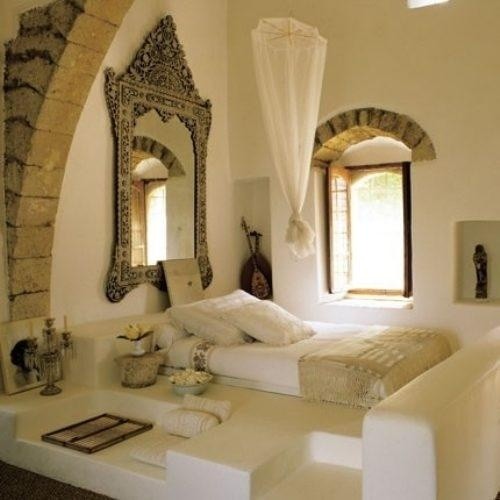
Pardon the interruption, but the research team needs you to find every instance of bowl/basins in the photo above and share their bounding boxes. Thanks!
[169,372,213,396]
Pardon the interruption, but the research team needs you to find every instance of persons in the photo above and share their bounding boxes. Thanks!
[10,339,41,386]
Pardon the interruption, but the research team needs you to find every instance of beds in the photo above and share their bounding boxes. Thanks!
[150,309,458,407]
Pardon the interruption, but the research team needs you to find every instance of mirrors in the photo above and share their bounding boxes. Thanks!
[103,15,212,303]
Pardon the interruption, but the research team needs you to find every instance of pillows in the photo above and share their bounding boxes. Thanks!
[224,299,310,345]
[166,289,264,346]
